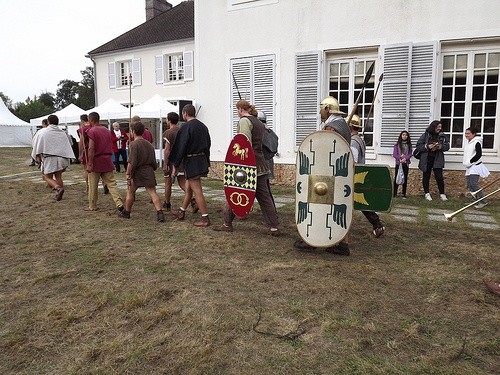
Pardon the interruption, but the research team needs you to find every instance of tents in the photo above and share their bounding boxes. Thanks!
[0,94,181,161]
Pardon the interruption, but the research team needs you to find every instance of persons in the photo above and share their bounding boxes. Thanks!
[416,120,450,201]
[462,127,490,209]
[393,131,412,200]
[29,97,385,256]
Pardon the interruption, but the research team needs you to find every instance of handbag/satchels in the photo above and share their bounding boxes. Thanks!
[396,162,405,185]
[414,133,426,159]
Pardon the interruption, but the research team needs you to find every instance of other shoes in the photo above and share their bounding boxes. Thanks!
[85,207,93,211]
[170,208,185,220]
[475,202,488,208]
[119,209,130,218]
[294,241,316,249]
[191,199,198,214]
[213,224,233,231]
[371,222,384,236]
[193,216,210,226]
[29,164,35,166]
[162,201,171,212]
[157,210,164,222]
[439,194,448,202]
[57,188,64,201]
[117,205,123,214]
[104,184,109,194]
[326,244,350,256]
[267,229,281,236]
[425,193,433,201]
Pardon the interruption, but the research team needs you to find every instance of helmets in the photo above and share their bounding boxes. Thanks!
[320,97,345,115]
[345,114,362,128]
[257,111,266,121]
